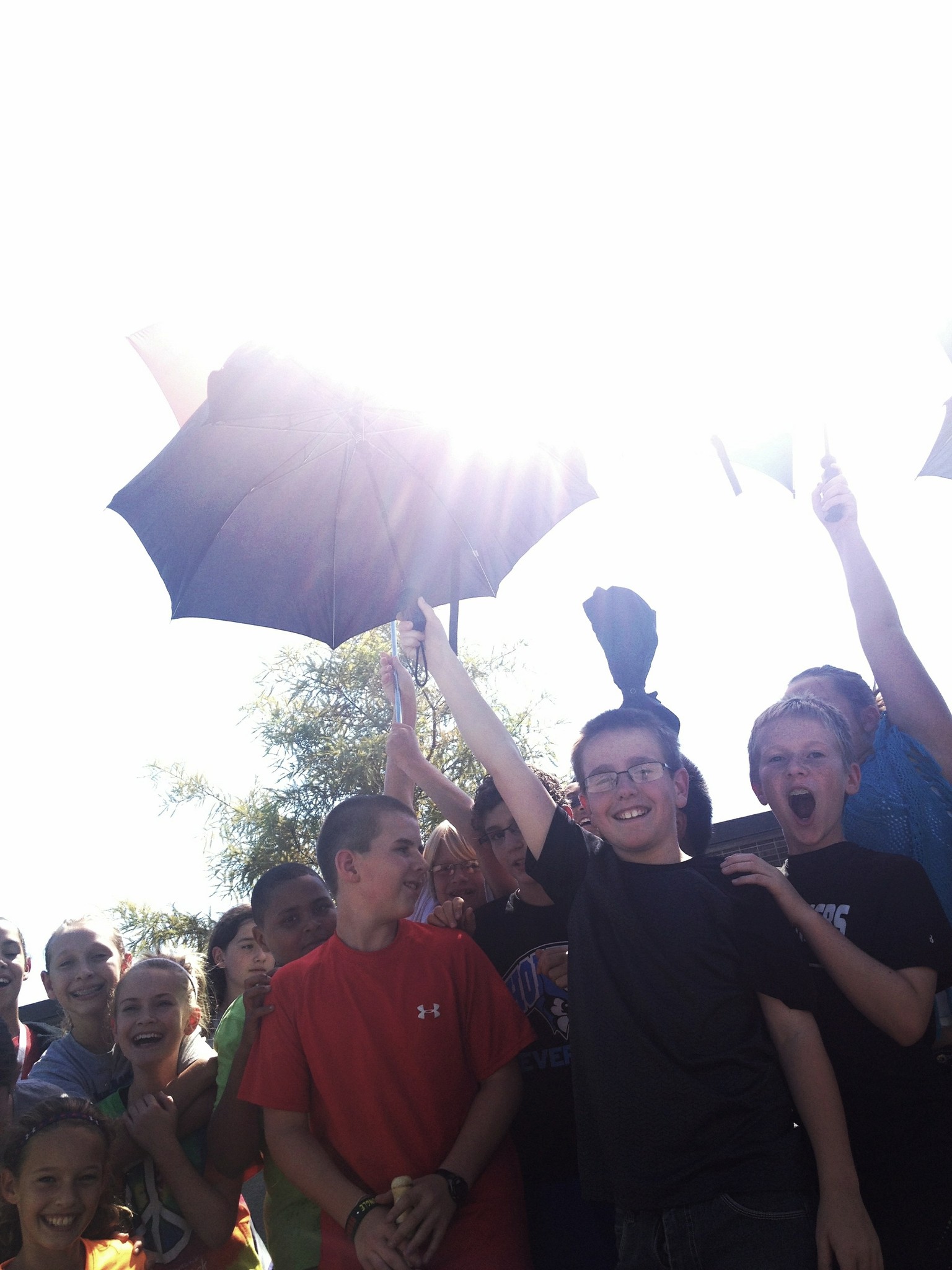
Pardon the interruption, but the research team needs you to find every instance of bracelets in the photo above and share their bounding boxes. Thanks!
[345,1197,378,1242]
[355,1194,368,1205]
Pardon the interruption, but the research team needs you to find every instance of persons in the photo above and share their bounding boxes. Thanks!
[0,457,952,1270]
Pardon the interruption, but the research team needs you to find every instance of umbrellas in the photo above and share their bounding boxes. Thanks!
[115,342,601,688]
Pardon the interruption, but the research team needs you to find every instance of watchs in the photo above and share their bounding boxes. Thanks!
[432,1168,469,1207]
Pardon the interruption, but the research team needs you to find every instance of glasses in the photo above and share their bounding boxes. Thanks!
[567,796,581,808]
[583,760,673,793]
[429,859,481,878]
[478,820,522,850]
